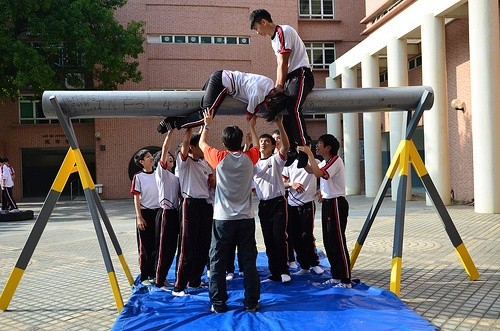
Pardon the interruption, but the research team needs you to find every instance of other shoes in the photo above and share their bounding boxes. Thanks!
[159,116,177,134]
[246,305,259,312]
[211,304,218,313]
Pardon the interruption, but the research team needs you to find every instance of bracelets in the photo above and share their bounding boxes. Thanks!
[204,125,211,130]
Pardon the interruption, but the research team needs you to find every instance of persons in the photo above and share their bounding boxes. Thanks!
[131,130,352,297]
[199,107,260,315]
[249,9,314,168]
[157,70,290,135]
[0,158,19,212]
